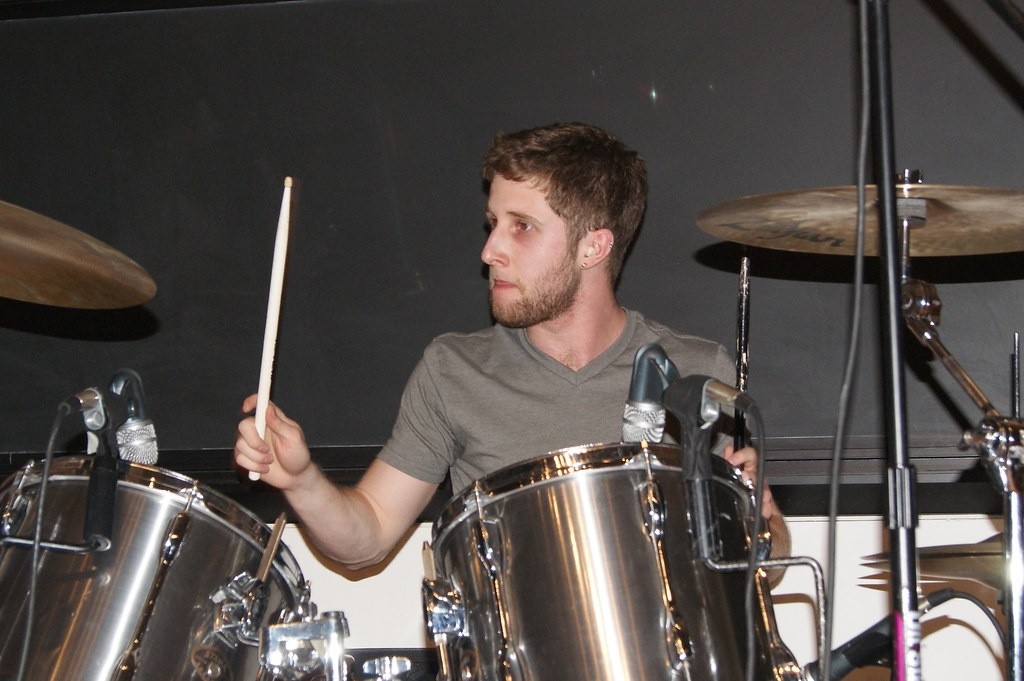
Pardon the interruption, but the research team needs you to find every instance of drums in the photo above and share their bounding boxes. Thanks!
[421,437,804,681]
[0,453,313,681]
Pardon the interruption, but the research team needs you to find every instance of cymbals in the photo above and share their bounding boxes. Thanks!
[859,525,1009,593]
[693,181,1024,259]
[0,195,161,313]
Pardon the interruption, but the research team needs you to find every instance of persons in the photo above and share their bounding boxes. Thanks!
[233,121,791,588]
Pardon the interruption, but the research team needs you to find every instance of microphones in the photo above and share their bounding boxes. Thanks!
[58,367,159,467]
[802,586,955,681]
[622,344,755,444]
